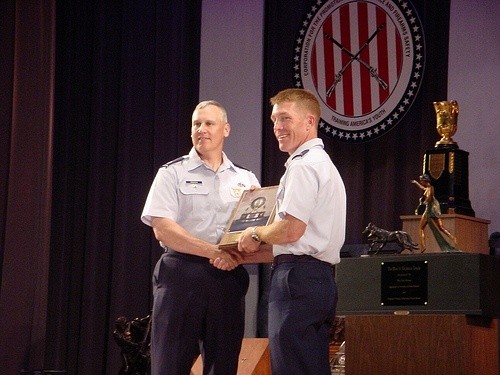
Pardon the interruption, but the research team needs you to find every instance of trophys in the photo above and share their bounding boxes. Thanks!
[413,98,478,216]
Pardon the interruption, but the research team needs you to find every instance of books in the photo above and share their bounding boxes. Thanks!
[218,180,280,251]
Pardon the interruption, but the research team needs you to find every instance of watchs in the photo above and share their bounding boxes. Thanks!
[251,227,260,242]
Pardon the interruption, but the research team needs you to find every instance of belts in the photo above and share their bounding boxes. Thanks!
[163,246,172,253]
[273,254,329,267]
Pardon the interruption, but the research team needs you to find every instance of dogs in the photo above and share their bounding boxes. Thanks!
[361,222,421,255]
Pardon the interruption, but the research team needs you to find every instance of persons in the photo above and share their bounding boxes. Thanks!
[217,89,345,375]
[409,173,460,255]
[139,102,251,375]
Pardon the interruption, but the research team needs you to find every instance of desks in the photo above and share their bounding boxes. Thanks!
[344,314,500,375]
